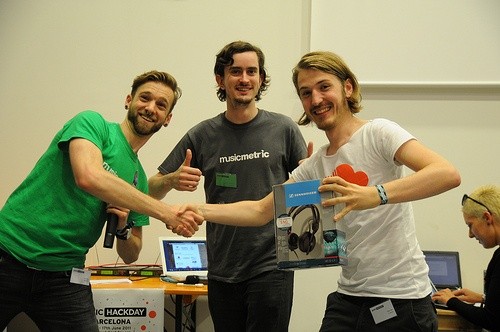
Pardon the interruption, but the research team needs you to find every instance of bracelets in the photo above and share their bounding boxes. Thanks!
[374,184,388,206]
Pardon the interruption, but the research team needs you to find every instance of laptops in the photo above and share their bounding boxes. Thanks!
[158,236,209,282]
[422,251,475,310]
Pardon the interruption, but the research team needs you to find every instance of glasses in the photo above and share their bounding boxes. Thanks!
[462,194,492,215]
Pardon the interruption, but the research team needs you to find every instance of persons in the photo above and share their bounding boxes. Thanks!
[166,51,463,332]
[0,69,205,332]
[146,40,315,332]
[431,185,500,332]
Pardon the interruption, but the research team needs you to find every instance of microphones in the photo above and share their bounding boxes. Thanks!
[103,206,120,248]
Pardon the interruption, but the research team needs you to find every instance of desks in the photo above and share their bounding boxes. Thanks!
[90,276,208,332]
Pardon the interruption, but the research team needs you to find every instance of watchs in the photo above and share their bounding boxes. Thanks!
[115,223,133,241]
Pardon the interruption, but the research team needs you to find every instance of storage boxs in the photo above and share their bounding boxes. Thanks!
[272,179,347,271]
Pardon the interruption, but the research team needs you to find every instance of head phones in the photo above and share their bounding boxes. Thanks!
[287,203,319,256]
